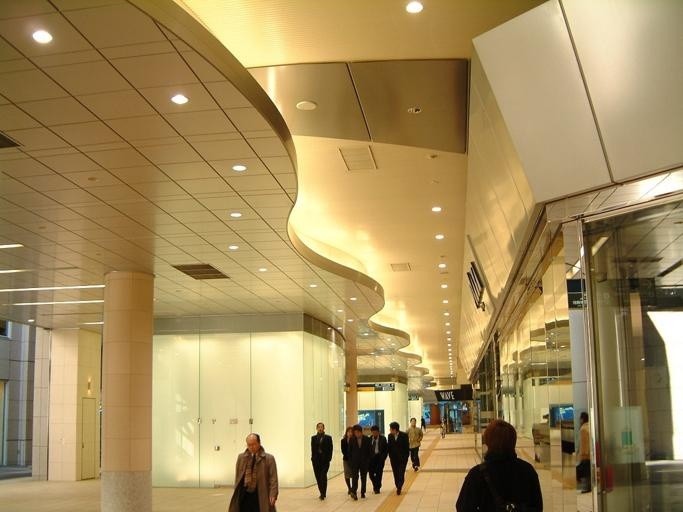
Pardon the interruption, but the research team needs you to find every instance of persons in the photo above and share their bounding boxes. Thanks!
[421,416,426,430]
[341,427,354,494]
[311,423,333,500]
[228,433,278,512]
[577,412,591,493]
[369,425,388,494]
[348,425,371,500]
[388,422,409,495]
[406,418,423,471]
[456,419,543,512]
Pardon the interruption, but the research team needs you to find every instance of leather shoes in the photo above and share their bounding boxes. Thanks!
[348,488,380,501]
[396,488,400,495]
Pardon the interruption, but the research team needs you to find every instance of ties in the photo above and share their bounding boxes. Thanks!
[251,454,256,474]
[372,439,375,453]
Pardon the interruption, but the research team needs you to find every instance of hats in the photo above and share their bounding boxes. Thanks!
[484,419,516,449]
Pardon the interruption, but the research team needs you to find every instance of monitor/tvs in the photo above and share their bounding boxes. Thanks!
[550,405,574,426]
[358,410,384,435]
